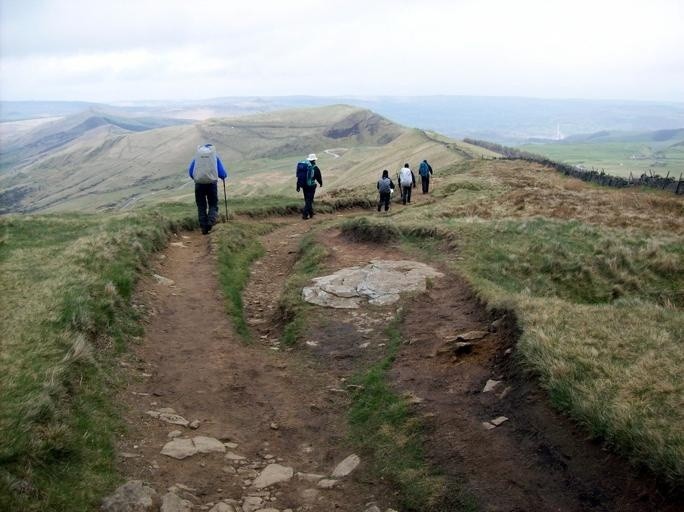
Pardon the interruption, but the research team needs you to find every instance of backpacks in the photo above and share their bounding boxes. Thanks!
[378,177,390,195]
[192,144,218,186]
[294,160,318,188]
[398,168,412,188]
[418,161,430,177]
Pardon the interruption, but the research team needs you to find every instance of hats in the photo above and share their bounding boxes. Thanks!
[305,153,319,161]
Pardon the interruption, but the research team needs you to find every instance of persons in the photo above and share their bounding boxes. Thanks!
[418,159,433,195]
[376,170,395,212]
[187,143,227,235]
[397,163,416,205]
[295,153,323,220]
